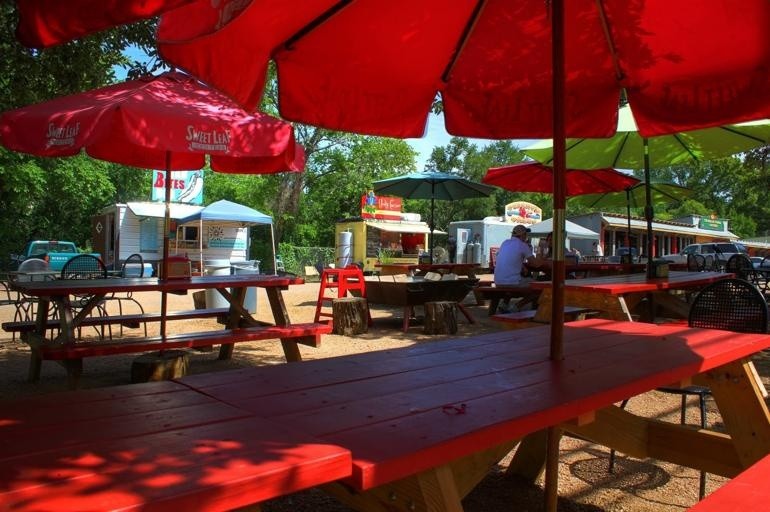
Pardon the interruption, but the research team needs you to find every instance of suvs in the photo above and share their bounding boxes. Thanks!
[662,243,747,271]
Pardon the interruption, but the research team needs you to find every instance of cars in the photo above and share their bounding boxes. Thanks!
[748,257,770,271]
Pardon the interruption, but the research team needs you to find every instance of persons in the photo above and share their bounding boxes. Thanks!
[530,230,578,311]
[494,224,546,313]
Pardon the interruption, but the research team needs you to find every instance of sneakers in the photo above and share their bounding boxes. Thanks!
[499,302,519,313]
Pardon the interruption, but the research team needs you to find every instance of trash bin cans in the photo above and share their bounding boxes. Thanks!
[231,260,261,314]
[205,259,231,309]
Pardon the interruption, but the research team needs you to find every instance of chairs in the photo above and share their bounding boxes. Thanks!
[0,252,147,341]
[686,251,770,303]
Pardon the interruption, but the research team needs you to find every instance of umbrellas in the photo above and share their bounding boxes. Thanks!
[480,159,641,196]
[19,0,770,512]
[567,180,697,262]
[371,169,497,264]
[1,64,308,353]
[517,101,770,325]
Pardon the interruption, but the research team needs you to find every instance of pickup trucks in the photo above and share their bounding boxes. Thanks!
[8,239,102,276]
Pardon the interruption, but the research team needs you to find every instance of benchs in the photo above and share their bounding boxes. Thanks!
[0,263,769,512]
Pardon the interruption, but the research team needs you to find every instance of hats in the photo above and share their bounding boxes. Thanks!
[512,225,531,236]
